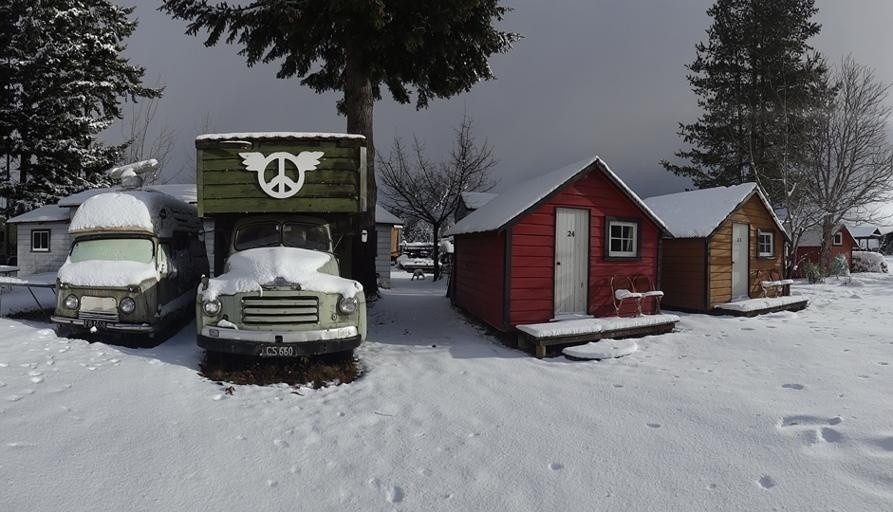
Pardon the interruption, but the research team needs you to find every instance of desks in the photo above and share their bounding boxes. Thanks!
[0,278,56,323]
[0,265,20,290]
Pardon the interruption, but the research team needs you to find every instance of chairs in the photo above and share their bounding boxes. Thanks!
[757,270,782,299]
[771,270,794,298]
[611,273,646,319]
[634,272,664,317]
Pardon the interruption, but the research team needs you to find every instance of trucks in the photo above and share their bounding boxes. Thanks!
[194,131,368,380]
[49,188,209,345]
